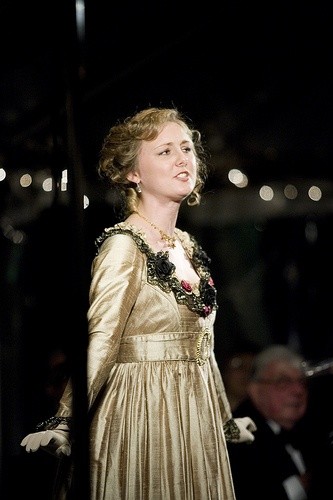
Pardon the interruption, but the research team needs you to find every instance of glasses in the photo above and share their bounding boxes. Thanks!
[250,373,308,387]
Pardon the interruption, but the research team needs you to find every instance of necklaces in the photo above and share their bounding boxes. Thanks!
[134,210,176,248]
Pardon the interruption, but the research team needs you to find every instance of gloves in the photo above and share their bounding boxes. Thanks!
[20,430,71,457]
[222,417,257,443]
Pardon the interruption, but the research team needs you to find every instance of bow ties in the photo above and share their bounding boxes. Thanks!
[280,427,304,441]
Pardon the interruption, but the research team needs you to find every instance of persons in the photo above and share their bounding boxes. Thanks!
[20,109,258,500]
[223,345,326,500]
[218,342,260,412]
